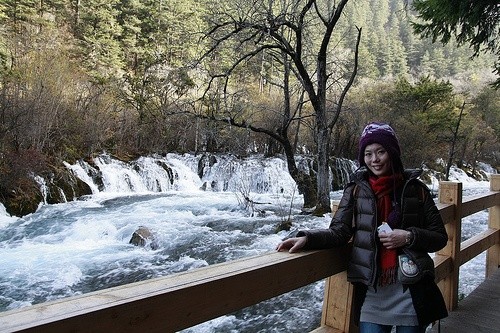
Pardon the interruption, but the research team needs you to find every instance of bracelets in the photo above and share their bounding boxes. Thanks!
[405,232,412,245]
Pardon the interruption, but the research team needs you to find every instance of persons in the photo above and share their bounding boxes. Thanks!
[276,123,448,333]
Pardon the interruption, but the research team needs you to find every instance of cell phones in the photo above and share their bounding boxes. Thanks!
[377,223,392,235]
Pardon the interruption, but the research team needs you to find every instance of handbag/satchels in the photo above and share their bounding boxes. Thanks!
[397,247,436,285]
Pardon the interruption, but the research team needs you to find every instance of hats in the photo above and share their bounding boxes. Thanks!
[355,120,405,222]
